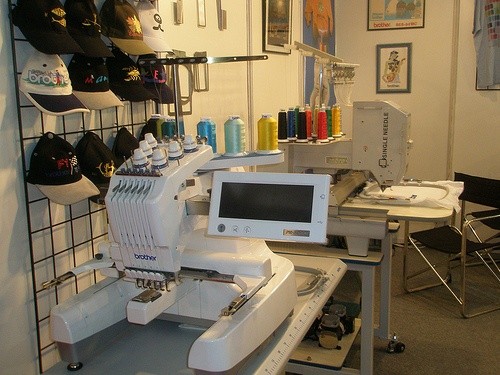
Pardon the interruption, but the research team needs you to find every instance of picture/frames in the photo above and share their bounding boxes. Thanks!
[367,0,425,31]
[262,0,292,54]
[376,42,412,93]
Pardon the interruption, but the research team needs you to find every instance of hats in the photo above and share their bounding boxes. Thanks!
[7,0,175,205]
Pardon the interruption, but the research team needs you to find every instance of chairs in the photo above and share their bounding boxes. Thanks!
[398,170,500,318]
